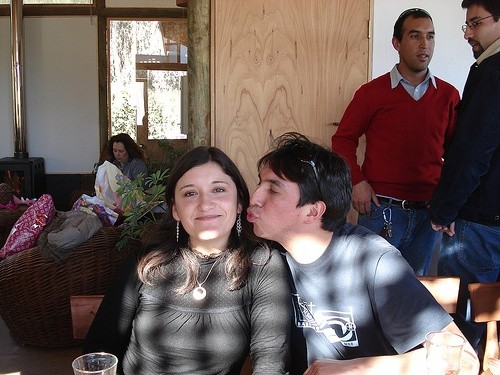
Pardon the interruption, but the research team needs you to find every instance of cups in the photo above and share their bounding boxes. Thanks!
[72,352,118,375]
[426,329,465,374]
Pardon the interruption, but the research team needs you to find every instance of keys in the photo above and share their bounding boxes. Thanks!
[382,222,393,239]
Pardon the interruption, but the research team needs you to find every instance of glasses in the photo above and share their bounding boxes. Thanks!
[280,145,324,203]
[461,13,500,33]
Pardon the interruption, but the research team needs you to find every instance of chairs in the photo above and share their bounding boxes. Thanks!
[0,225,142,348]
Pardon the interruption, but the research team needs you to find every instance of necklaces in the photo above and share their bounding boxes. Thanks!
[184,247,225,303]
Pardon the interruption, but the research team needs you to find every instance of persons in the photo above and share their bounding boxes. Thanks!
[78,144,297,374]
[99,133,148,186]
[331,8,460,277]
[244,130,481,375]
[430,0,500,351]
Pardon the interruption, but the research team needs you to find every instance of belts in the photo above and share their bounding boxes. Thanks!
[376,197,430,210]
